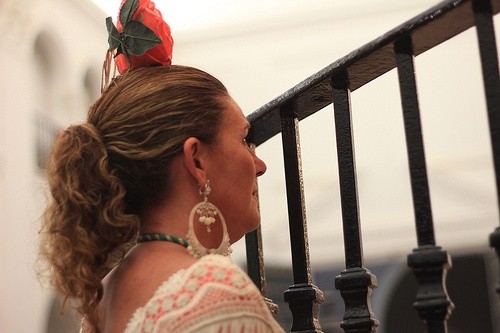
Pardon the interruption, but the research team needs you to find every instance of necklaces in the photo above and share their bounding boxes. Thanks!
[135,232,190,249]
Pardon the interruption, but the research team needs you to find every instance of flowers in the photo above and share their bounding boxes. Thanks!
[105,0,174,74]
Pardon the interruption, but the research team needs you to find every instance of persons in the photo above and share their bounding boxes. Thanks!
[38,62,284,332]
